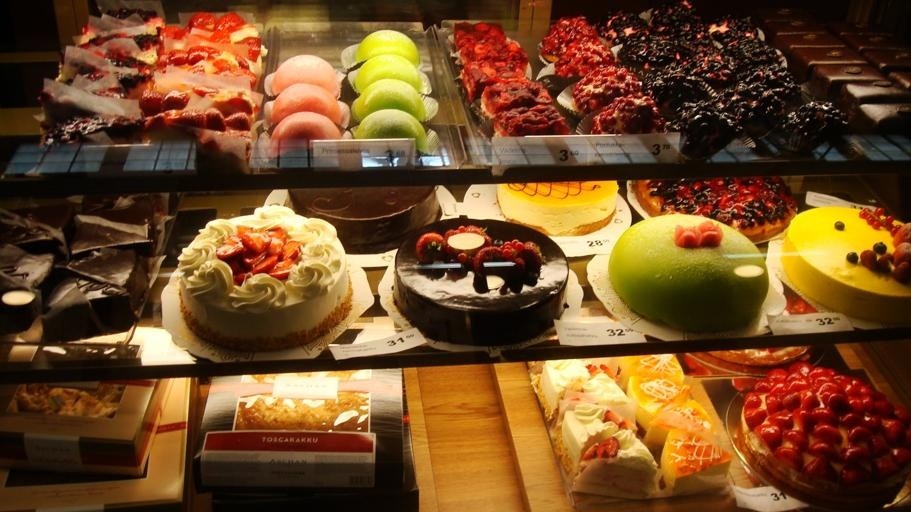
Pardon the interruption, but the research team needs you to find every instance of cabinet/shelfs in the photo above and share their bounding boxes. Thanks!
[0,0,911,512]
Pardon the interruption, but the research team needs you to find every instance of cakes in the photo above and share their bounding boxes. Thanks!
[780,206,910,324]
[750,0,911,134]
[735,362,910,509]
[354,30,420,67]
[527,353,733,499]
[288,185,441,254]
[270,83,343,126]
[355,109,427,151]
[271,55,337,96]
[177,204,353,353]
[608,213,768,333]
[355,79,427,122]
[635,175,796,243]
[354,55,423,92]
[32,7,268,169]
[688,343,812,377]
[496,180,619,236]
[454,15,666,136]
[0,192,163,344]
[268,111,341,159]
[393,219,570,346]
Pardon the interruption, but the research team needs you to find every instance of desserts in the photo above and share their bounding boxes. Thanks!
[587,0,849,162]
